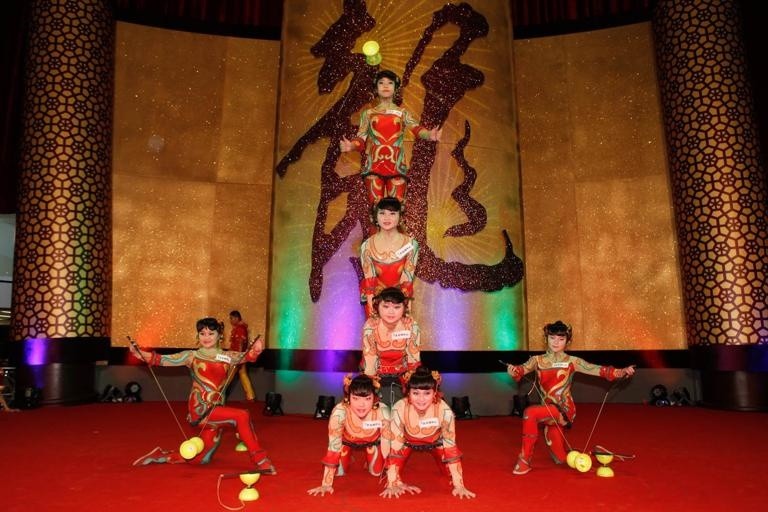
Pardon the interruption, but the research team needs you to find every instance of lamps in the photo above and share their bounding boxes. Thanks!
[313,394,336,418]
[261,392,285,417]
[97,382,142,403]
[451,394,528,420]
[648,385,689,407]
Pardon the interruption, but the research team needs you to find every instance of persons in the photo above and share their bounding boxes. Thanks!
[357,195,422,321]
[355,285,422,408]
[226,308,255,403]
[504,318,638,474]
[336,67,445,238]
[304,373,423,500]
[379,364,478,500]
[126,315,280,477]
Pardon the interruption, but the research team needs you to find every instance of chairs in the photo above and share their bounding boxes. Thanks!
[0,368,11,412]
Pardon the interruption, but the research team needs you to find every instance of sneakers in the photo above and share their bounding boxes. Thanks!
[255,457,278,476]
[595,445,625,463]
[132,446,168,467]
[512,459,533,475]
